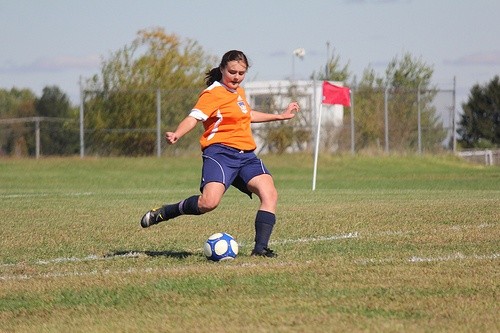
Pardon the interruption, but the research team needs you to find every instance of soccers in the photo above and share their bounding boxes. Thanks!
[205,233,239,262]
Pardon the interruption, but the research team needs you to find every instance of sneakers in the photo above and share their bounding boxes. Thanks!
[251,243,278,259]
[141,204,169,228]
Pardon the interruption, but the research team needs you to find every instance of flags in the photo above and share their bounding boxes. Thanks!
[321,80,351,107]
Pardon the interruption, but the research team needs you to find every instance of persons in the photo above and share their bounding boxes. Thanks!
[141,50,300,258]
